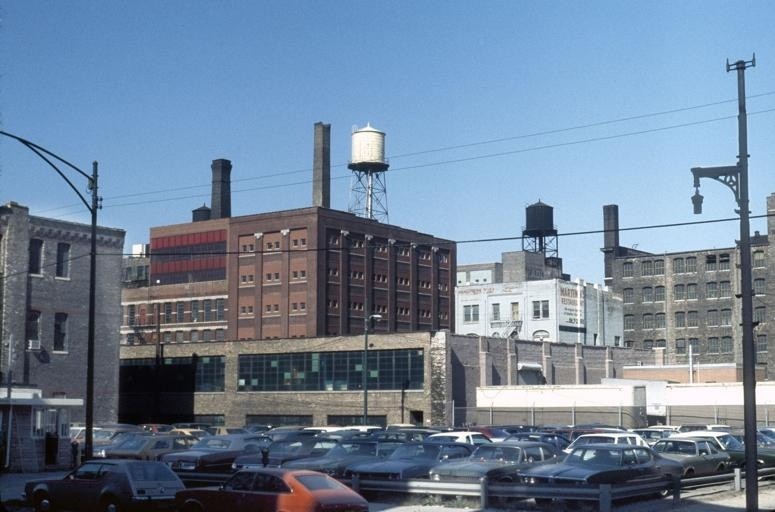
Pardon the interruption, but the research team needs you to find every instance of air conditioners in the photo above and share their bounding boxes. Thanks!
[27,340,41,350]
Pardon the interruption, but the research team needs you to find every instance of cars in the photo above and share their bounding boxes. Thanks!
[173,467,370,512]
[515,443,686,509]
[69,422,775,502]
[23,459,188,512]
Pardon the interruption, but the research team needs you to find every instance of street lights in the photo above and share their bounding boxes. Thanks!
[1,132,104,459]
[689,163,759,511]
[364,313,384,424]
